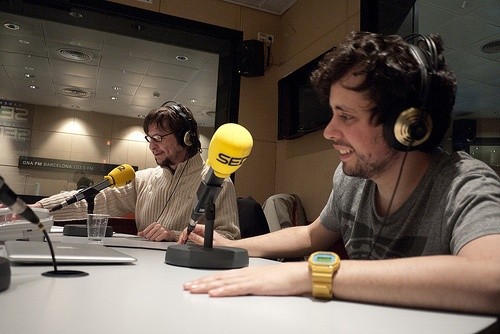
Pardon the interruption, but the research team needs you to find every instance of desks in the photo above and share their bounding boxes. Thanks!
[0,233,497,334]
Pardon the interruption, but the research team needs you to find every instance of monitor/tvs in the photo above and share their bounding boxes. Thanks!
[277,47,337,141]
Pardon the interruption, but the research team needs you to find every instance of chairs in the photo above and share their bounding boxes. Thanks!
[238,196,271,238]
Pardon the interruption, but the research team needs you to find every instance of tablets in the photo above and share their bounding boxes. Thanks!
[4,241,137,263]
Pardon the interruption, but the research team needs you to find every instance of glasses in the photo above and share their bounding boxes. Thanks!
[144,131,177,142]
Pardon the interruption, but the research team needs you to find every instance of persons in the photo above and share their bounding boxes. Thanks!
[0,101,240,242]
[75,177,94,190]
[176,31,500,317]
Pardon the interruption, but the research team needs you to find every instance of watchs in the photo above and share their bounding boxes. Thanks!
[308,252,340,300]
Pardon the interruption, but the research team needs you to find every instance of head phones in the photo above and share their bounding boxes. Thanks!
[163,101,199,149]
[381,32,438,152]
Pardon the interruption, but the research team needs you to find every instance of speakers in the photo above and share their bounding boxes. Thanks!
[231,40,265,78]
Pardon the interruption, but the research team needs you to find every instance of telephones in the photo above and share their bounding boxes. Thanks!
[0,207,54,242]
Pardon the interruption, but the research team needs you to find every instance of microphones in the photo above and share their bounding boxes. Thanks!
[0,176,45,230]
[187,123,254,233]
[51,164,135,212]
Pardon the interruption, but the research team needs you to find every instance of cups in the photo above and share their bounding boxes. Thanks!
[85,213,111,242]
